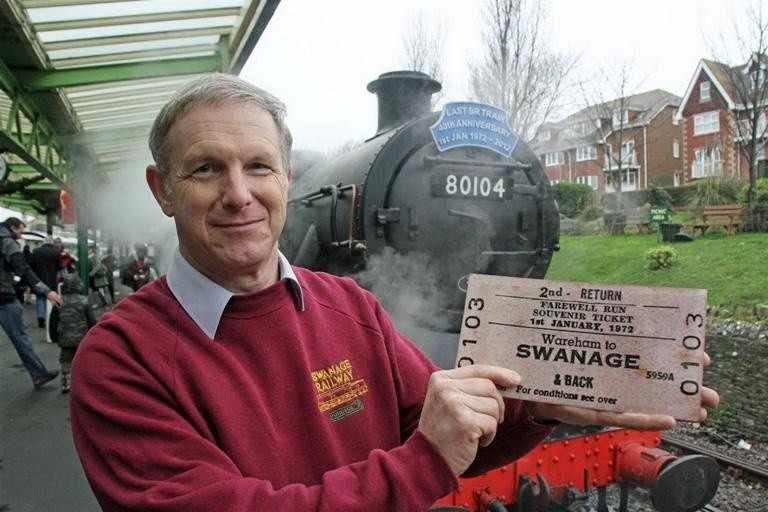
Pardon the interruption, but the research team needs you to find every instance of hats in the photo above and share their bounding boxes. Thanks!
[44,235,54,243]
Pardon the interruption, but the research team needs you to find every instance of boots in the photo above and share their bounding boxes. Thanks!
[61,371,71,393]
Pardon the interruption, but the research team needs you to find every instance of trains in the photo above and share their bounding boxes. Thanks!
[278,70,721,512]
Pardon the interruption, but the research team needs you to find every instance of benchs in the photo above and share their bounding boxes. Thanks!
[604,202,745,237]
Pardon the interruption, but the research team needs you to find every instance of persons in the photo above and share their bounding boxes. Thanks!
[48,274,97,393]
[69,71,722,510]
[1,217,65,386]
[14,238,159,343]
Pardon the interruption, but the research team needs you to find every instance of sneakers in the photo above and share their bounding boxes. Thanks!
[33,369,60,387]
[91,301,118,310]
[37,318,45,328]
